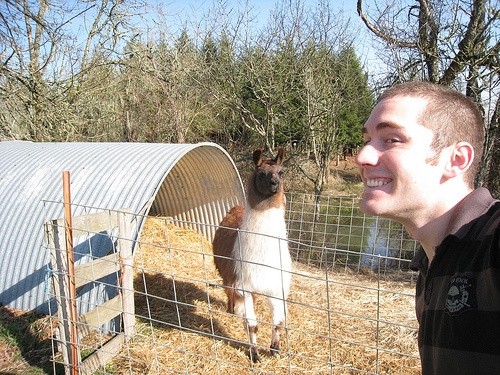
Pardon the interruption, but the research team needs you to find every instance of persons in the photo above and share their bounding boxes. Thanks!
[354,81,500,375]
